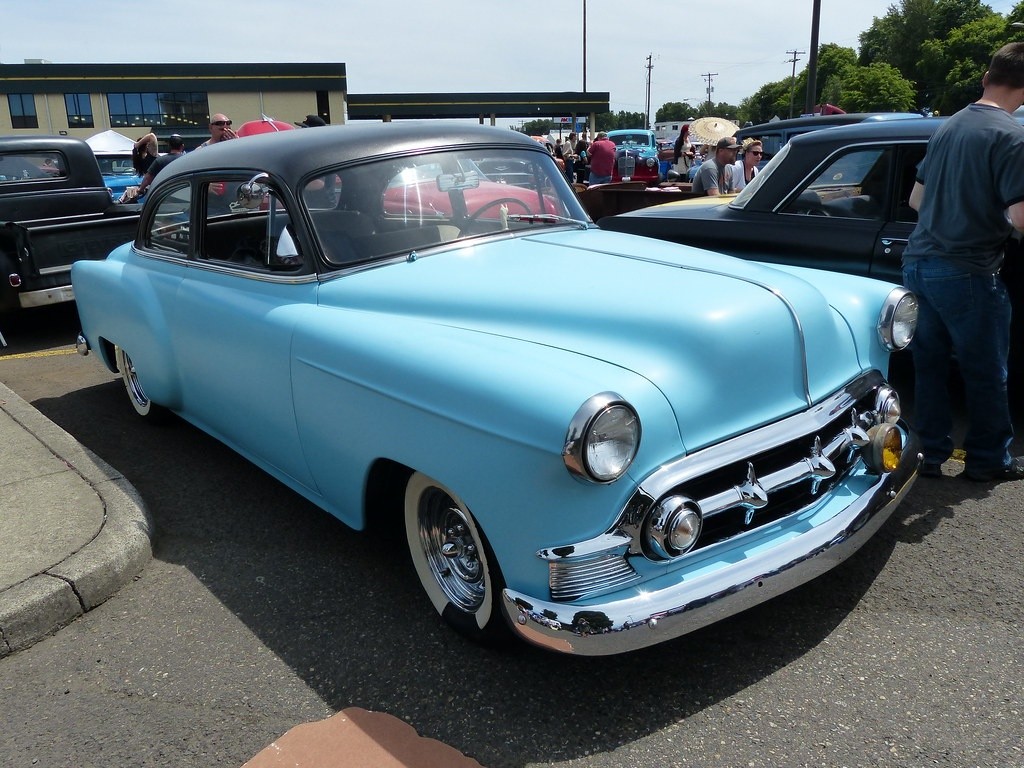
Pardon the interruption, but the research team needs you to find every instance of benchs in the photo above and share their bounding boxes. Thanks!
[178,209,375,264]
[796,189,921,222]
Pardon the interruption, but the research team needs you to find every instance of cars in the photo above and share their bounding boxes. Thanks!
[575,113,1024,287]
[0,120,923,655]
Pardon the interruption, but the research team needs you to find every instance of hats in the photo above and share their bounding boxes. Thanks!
[717,138,741,149]
[295,114,326,128]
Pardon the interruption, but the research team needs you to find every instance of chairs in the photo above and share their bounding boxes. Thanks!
[355,225,466,259]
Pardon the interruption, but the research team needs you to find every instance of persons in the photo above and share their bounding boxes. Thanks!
[293,114,335,209]
[692,136,741,195]
[724,138,763,193]
[546,132,615,186]
[45,158,52,165]
[700,144,715,160]
[195,113,239,216]
[107,132,184,203]
[901,43,1024,482]
[674,124,694,182]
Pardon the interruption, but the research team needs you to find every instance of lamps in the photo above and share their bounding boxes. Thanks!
[59,131,67,135]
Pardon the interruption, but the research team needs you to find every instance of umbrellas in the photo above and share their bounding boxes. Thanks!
[689,117,741,146]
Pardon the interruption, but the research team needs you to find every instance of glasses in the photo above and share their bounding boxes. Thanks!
[748,151,764,156]
[211,120,233,126]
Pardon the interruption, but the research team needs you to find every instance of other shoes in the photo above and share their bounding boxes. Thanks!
[966,455,1023,480]
[917,461,942,475]
